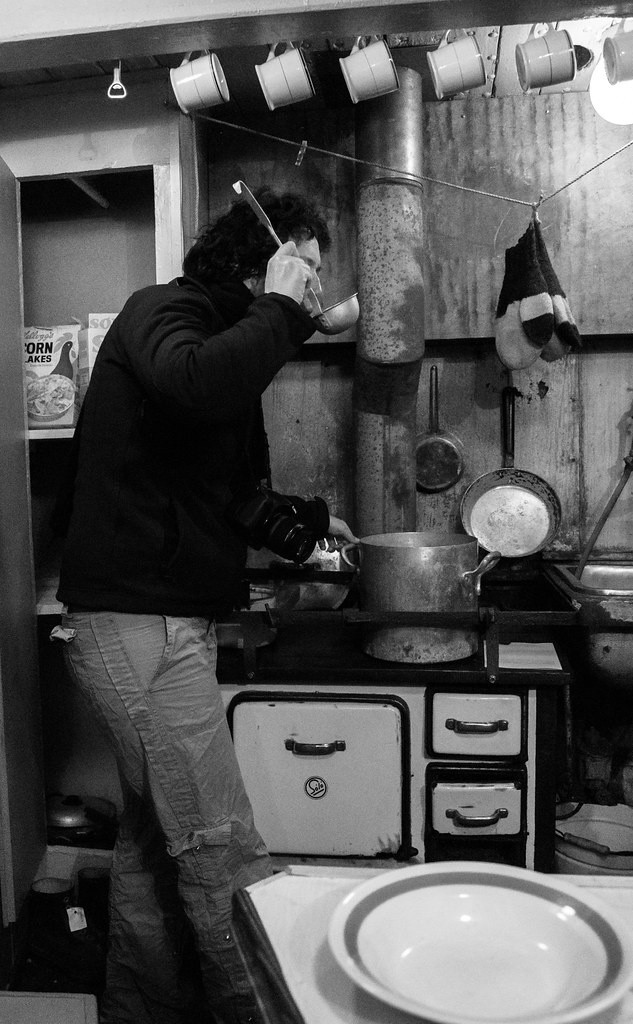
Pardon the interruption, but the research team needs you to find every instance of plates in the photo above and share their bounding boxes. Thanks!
[328,857,633,1024]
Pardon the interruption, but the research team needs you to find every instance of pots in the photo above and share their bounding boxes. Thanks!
[459,386,563,559]
[231,585,280,650]
[338,529,502,665]
[414,365,466,492]
[252,536,351,608]
[45,791,126,844]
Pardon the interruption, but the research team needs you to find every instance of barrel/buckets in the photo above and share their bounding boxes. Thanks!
[553,799,633,877]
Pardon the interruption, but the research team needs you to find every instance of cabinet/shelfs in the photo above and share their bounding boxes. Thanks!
[29,427,79,614]
[217,683,537,865]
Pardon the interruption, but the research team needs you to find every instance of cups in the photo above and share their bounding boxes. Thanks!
[337,30,401,104]
[426,27,487,100]
[169,44,231,114]
[602,17,633,86]
[253,38,317,112]
[514,22,578,92]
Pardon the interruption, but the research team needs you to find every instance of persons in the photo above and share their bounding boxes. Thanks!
[56,190,361,1024]
